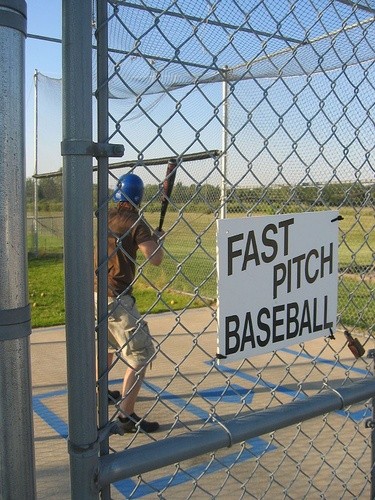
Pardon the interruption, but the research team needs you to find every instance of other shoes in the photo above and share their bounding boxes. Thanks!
[108,390,120,404]
[116,414,159,433]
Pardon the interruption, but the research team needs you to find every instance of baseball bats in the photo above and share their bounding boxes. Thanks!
[158,159,177,232]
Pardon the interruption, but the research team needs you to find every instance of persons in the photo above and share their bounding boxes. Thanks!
[91,174,166,433]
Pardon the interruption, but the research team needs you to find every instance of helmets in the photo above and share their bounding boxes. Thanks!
[112,174,144,203]
